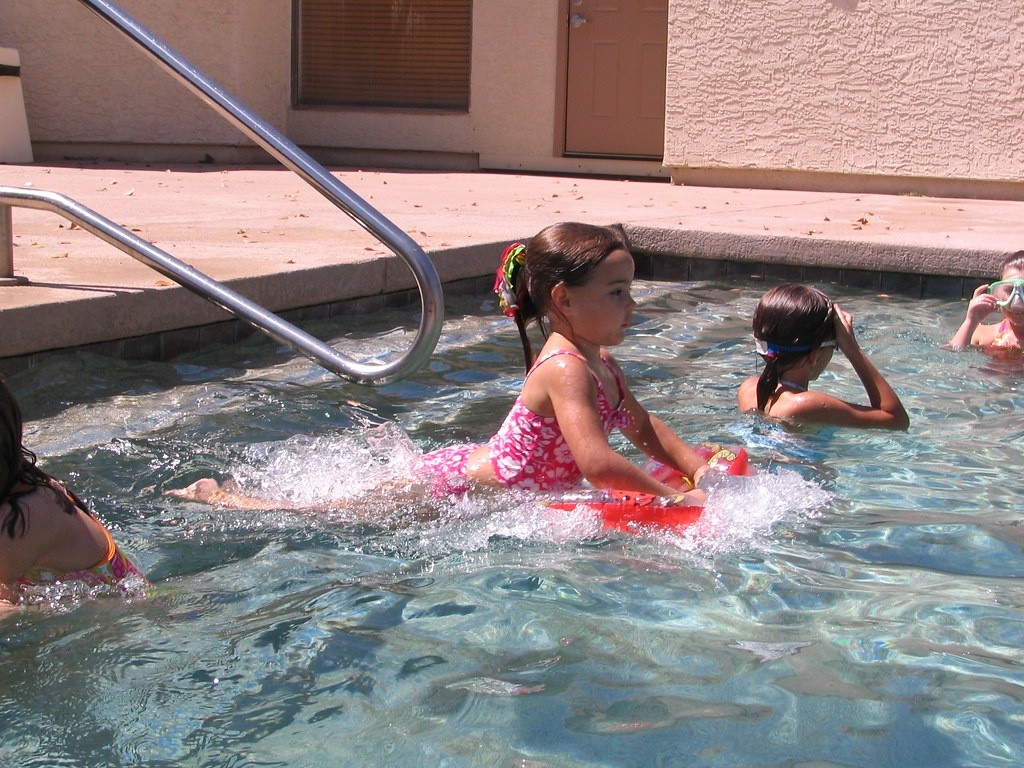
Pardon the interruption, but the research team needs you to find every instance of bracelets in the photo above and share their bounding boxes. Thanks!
[692,466,711,487]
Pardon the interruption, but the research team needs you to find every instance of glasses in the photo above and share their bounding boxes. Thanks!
[986,280,1024,309]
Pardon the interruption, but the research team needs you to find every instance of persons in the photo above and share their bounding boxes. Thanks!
[0,371,165,602]
[942,249,1024,381]
[164,222,721,530]
[708,282,910,540]
